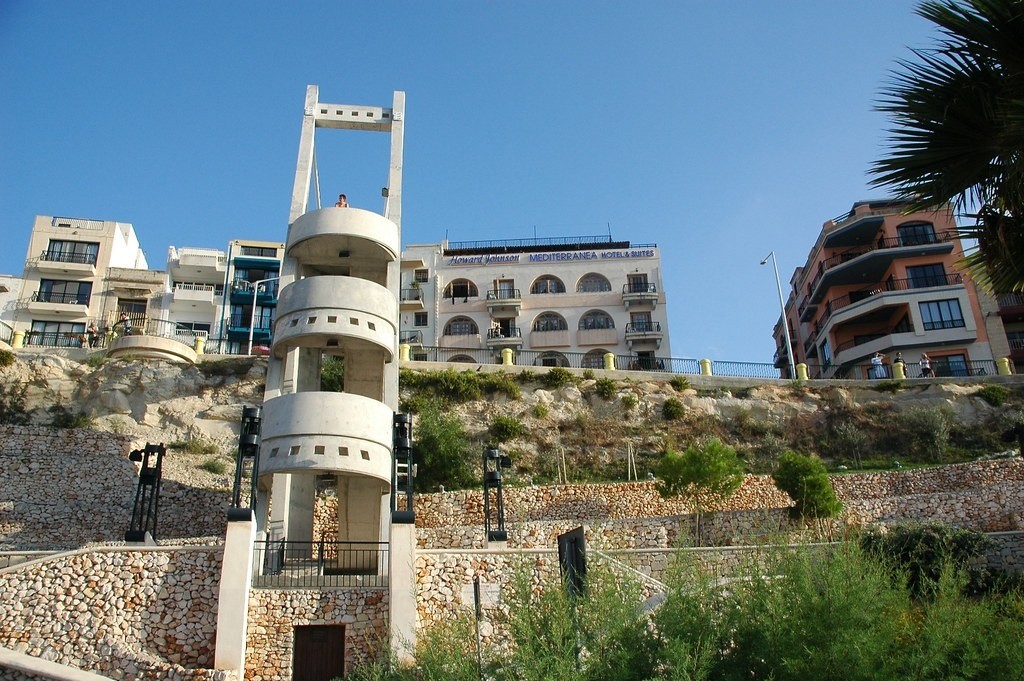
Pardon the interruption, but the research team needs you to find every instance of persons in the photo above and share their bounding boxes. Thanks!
[102,326,117,348]
[871,352,886,379]
[628,360,637,370]
[258,282,266,293]
[87,322,98,348]
[335,194,349,208]
[919,353,938,378]
[121,313,132,336]
[894,352,907,378]
[653,360,665,372]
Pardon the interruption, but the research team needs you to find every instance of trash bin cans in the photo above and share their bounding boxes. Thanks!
[195,338,206,355]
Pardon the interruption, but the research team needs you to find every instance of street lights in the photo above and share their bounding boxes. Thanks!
[759,250,797,379]
